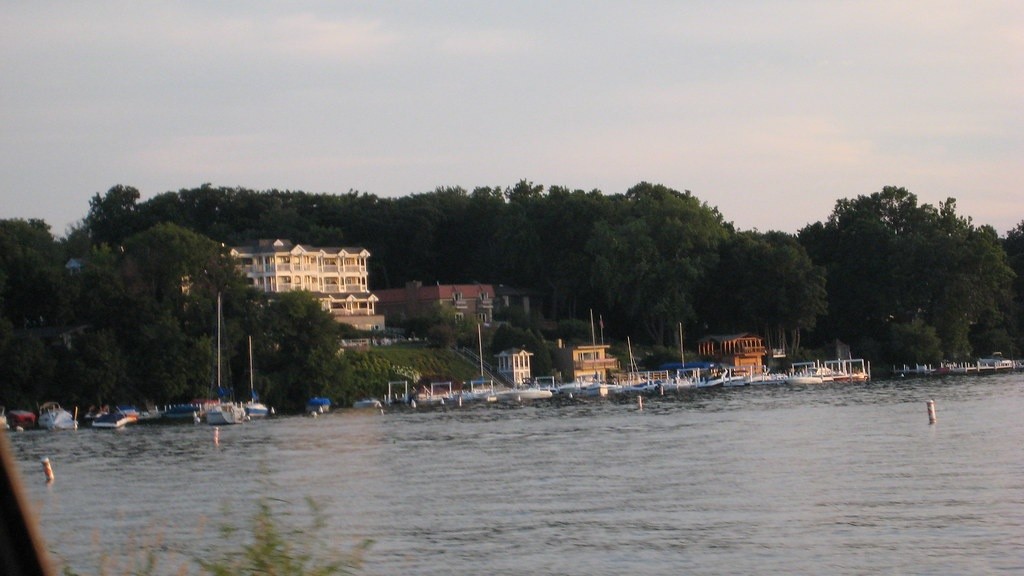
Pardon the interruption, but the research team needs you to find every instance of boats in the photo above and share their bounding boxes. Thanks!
[39,402,77,430]
[93,416,138,429]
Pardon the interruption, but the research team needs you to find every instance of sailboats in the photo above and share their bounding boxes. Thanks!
[354,309,873,410]
[206,292,267,424]
